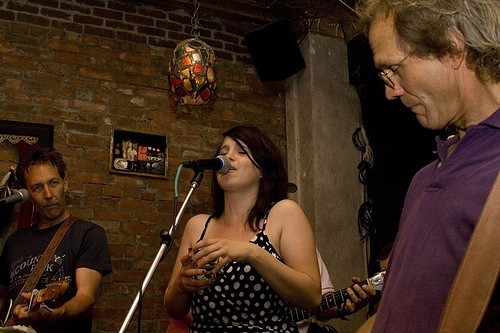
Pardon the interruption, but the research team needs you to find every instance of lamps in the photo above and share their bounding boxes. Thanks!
[168,0,217,113]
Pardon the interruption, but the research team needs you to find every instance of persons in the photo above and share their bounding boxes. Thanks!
[356,0,500,333]
[296,247,376,333]
[165,125,322,333]
[0,148,113,333]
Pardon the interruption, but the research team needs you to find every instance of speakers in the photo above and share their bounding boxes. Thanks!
[243,20,306,83]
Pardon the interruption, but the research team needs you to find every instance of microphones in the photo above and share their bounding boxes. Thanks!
[0,189,30,203]
[180,155,230,175]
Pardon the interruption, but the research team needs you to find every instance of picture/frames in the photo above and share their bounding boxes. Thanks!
[107,128,171,178]
[0,120,55,251]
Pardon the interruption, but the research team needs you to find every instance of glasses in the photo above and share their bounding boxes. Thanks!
[378,49,418,88]
